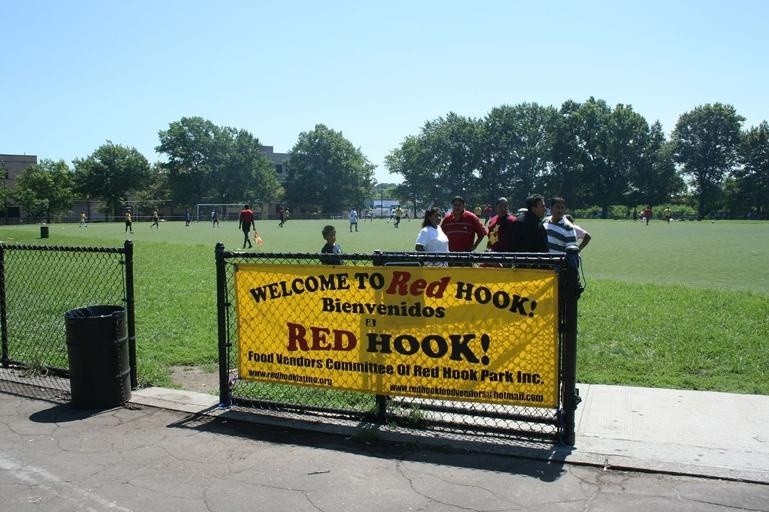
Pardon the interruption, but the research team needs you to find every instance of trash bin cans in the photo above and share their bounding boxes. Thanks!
[41,227,49,238]
[63,305,132,409]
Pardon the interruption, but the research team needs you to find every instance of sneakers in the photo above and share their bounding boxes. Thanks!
[248,245,253,249]
[243,247,246,249]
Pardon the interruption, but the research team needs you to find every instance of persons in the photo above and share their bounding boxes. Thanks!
[321,225,343,265]
[415,194,591,299]
[79,211,87,228]
[349,205,411,232]
[212,208,219,227]
[150,209,159,230]
[632,207,653,226]
[125,209,133,233]
[186,207,192,226]
[285,208,290,221]
[279,207,287,227]
[239,205,256,248]
[664,207,673,223]
